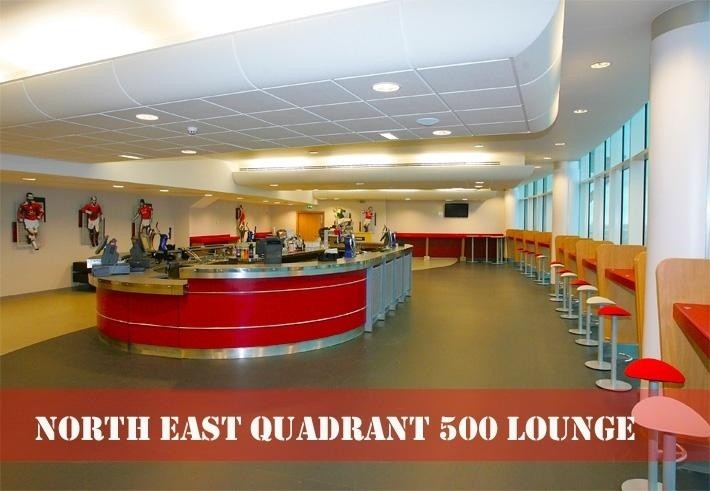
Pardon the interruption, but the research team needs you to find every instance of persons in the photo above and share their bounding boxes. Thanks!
[80,196,102,247]
[132,199,153,235]
[239,204,246,225]
[362,207,375,232]
[17,192,44,250]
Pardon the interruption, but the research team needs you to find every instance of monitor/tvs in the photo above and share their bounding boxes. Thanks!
[445,203,468,217]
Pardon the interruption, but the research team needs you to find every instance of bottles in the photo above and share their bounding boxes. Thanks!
[236,245,254,262]
[301,240,305,252]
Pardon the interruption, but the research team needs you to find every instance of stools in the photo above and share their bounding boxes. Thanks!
[469,234,708,491]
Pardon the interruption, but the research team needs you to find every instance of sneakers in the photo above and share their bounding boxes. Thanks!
[27,236,31,244]
[33,247,39,251]
[91,240,99,247]
